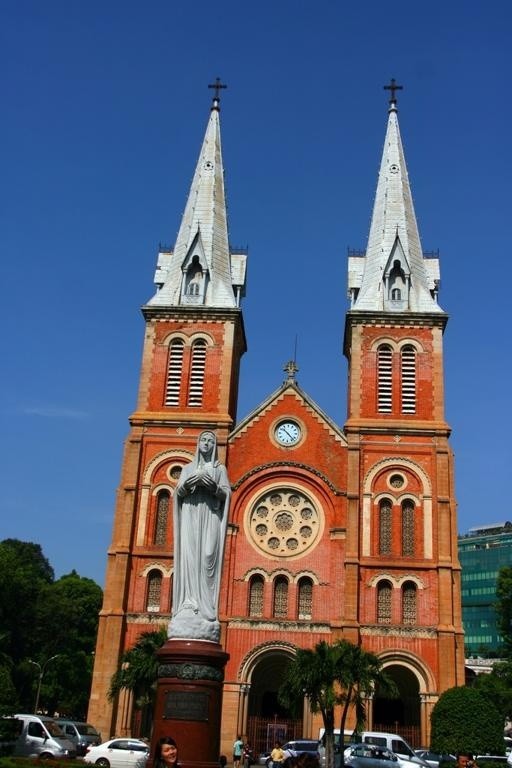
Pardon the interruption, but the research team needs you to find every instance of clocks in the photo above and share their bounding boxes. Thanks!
[272,417,303,447]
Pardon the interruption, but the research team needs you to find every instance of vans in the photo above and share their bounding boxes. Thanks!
[259,740,319,768]
[2,712,102,761]
[317,728,431,768]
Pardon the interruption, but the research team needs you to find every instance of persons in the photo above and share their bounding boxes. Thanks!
[454,750,479,768]
[151,736,181,768]
[271,742,284,768]
[283,751,321,768]
[37,707,60,717]
[233,736,257,768]
[167,430,230,642]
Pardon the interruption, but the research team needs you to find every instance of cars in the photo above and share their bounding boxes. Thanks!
[343,744,426,768]
[83,737,151,768]
[414,749,458,768]
